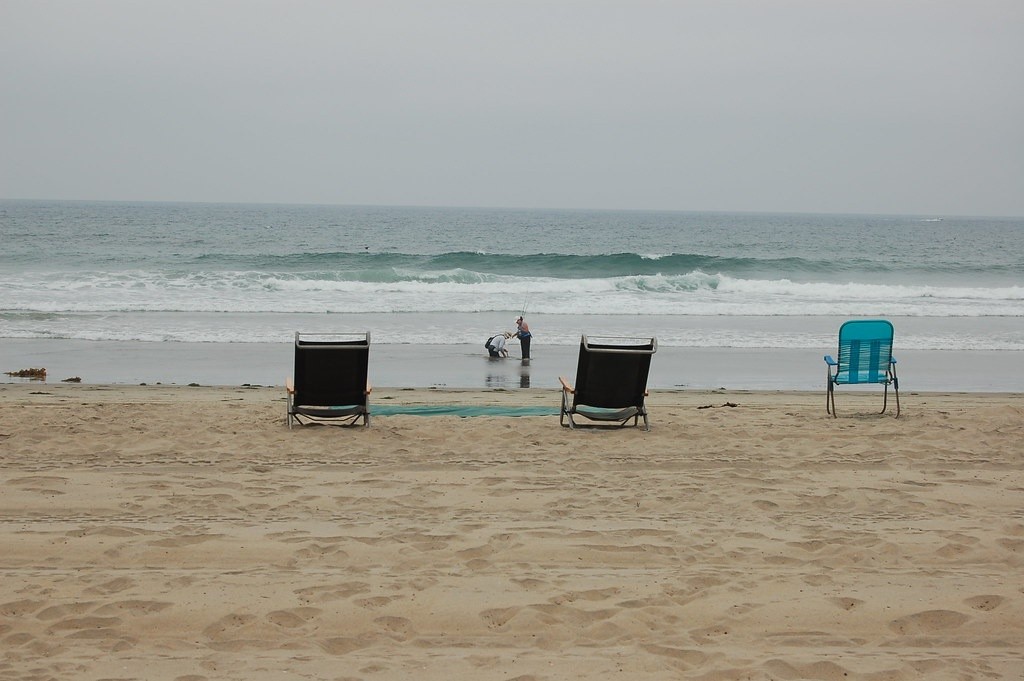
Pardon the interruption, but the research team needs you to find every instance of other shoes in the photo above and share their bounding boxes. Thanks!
[524,358,529,360]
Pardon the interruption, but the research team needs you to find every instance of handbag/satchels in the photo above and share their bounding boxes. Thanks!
[484,336,493,348]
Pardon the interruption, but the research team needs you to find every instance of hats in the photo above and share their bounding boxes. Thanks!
[505,330,513,336]
[513,315,520,323]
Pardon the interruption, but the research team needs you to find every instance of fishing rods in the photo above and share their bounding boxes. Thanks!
[518,274,532,337]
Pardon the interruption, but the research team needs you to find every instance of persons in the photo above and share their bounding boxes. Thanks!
[488,331,511,358]
[512,316,531,359]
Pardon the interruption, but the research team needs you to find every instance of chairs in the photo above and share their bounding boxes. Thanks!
[558,334,658,432]
[824,318,900,419]
[287,331,372,430]
